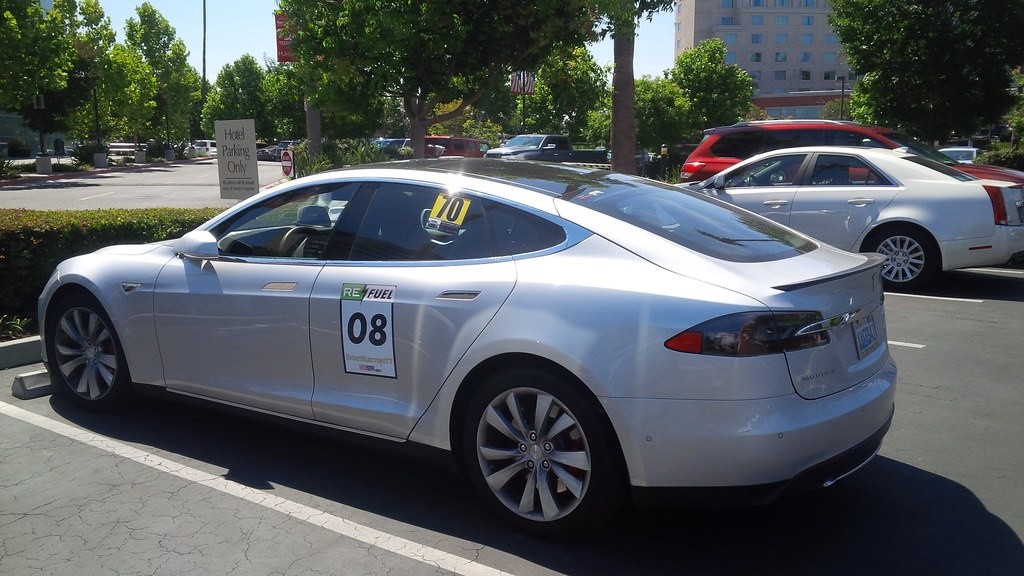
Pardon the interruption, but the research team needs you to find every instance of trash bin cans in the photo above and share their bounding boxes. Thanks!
[52,138,65,155]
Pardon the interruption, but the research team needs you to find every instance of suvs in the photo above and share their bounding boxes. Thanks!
[680,119,1024,187]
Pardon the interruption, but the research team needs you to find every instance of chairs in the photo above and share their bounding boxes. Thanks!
[371,191,429,260]
[827,158,852,184]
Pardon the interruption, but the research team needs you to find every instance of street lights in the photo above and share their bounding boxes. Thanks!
[835,74,846,120]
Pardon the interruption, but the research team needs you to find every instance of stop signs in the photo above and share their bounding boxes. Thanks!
[281,152,293,175]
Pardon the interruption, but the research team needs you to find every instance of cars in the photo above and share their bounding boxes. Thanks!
[37,159,900,547]
[256,141,302,162]
[666,145,1024,292]
[936,136,991,164]
[192,138,217,156]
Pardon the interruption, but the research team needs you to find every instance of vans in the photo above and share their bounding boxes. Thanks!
[372,135,491,161]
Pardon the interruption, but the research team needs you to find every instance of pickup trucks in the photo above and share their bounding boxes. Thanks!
[483,134,608,165]
